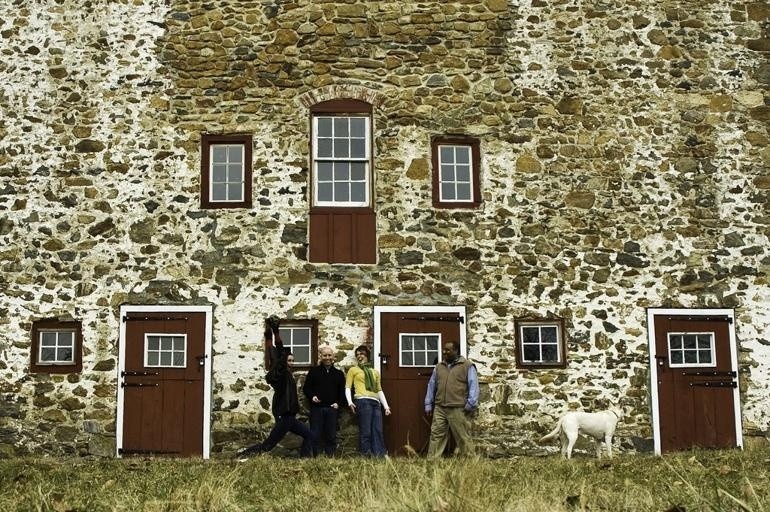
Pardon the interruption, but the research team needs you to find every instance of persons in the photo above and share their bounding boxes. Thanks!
[423,339,480,460]
[236,314,317,459]
[345,345,393,457]
[302,346,345,456]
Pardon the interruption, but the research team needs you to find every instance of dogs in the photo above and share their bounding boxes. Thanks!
[538,401,631,461]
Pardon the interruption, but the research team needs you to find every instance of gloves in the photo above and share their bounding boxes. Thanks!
[265,317,281,333]
